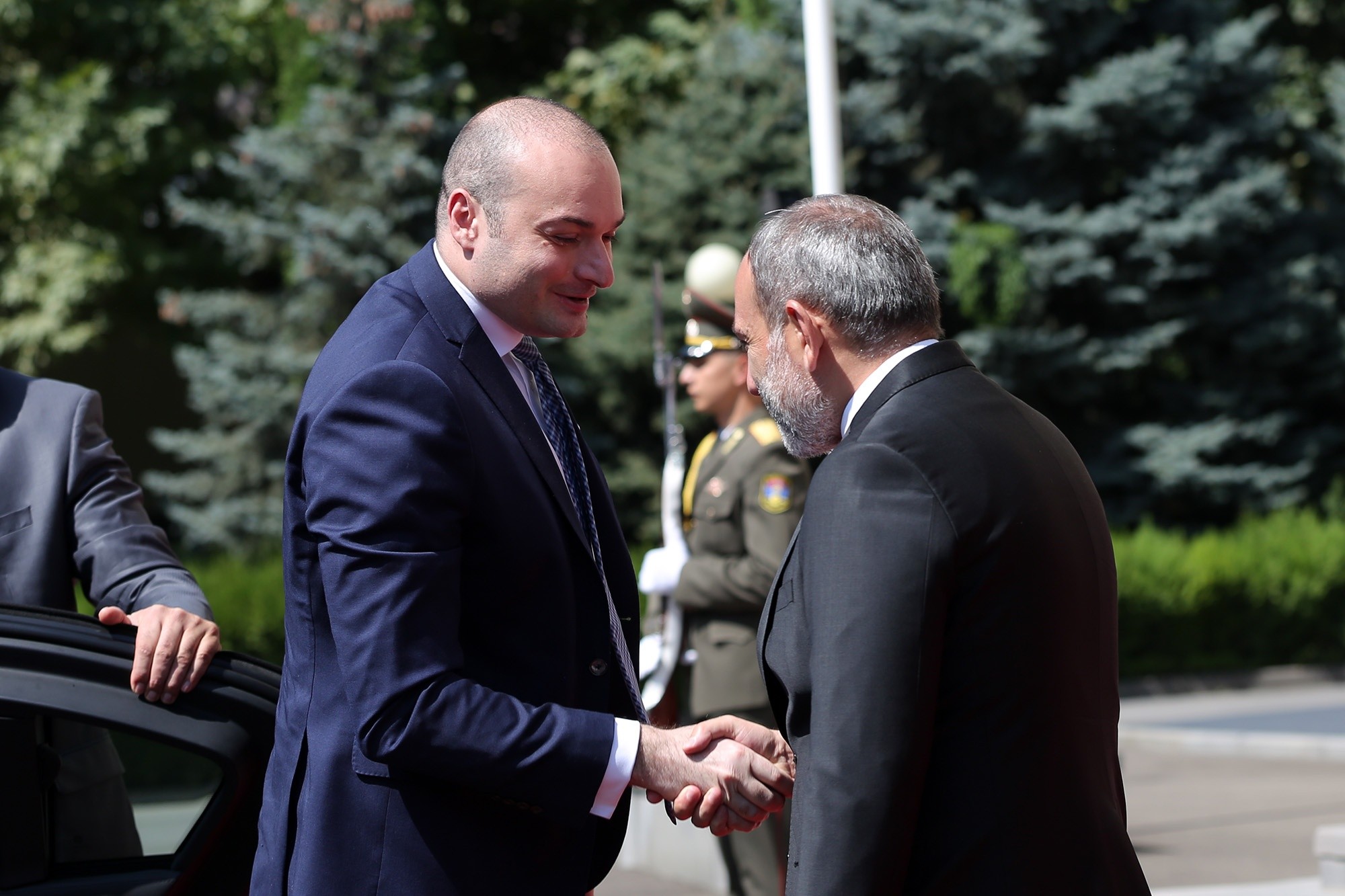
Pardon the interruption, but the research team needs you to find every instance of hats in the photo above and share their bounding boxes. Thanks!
[679,290,749,359]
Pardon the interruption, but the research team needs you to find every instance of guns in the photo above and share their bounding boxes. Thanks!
[637,259,689,710]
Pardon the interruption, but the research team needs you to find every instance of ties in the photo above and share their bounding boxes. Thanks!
[511,335,676,825]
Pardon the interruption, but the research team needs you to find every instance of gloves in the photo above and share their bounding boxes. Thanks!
[639,634,697,679]
[638,546,689,596]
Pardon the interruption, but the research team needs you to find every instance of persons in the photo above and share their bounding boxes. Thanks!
[644,192,1155,896]
[0,367,221,704]
[630,243,817,896]
[246,94,793,896]
